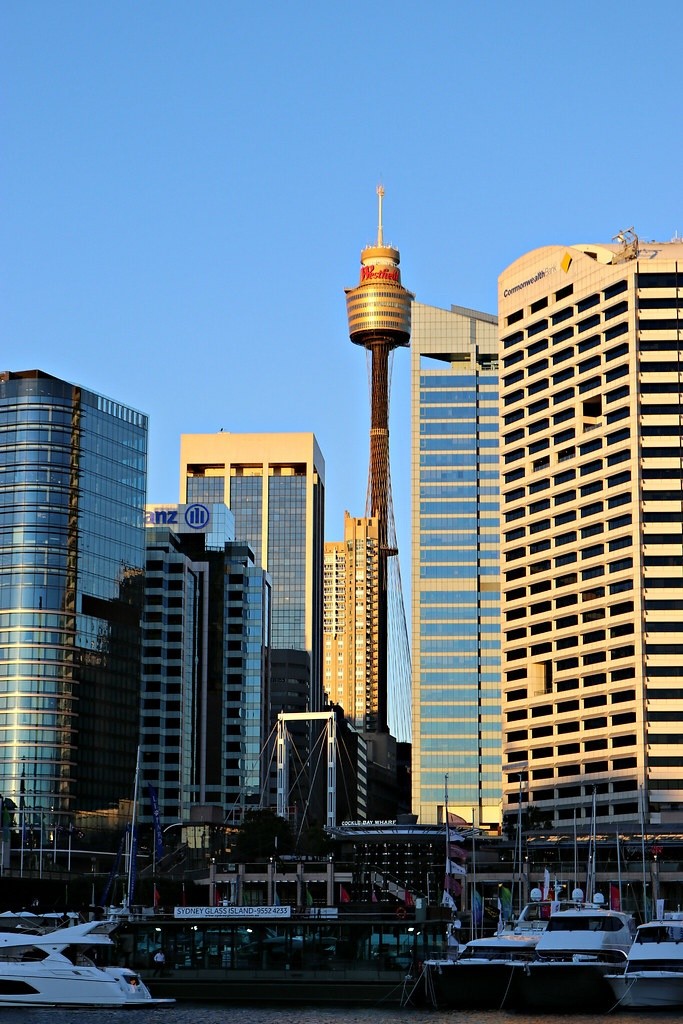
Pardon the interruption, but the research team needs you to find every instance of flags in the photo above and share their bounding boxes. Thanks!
[404,890,414,906]
[154,890,160,907]
[445,811,470,829]
[443,891,458,912]
[644,895,652,922]
[274,894,281,906]
[474,892,483,926]
[372,889,378,903]
[446,828,466,842]
[446,859,468,876]
[500,887,511,920]
[610,886,621,912]
[339,885,351,903]
[539,884,553,918]
[306,890,314,906]
[447,844,466,860]
[215,890,222,906]
[444,875,463,894]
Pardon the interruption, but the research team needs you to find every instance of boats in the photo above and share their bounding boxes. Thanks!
[0,686,683,1010]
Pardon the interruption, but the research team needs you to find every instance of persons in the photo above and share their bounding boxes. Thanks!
[152,948,165,978]
[59,910,70,928]
